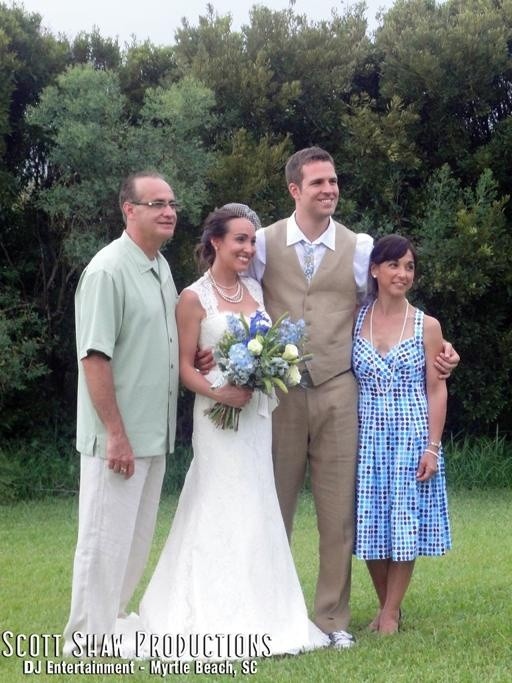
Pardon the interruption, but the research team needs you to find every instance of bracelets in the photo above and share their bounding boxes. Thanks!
[425,448,440,459]
[427,440,442,449]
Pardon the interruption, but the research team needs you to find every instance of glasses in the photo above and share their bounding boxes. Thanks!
[130,201,183,213]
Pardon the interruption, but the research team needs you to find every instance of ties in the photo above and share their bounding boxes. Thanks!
[302,244,315,283]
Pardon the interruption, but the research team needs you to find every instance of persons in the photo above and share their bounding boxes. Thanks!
[119,201,329,661]
[351,234,449,635]
[64,170,179,656]
[195,146,460,649]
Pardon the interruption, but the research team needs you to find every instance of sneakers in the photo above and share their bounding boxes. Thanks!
[329,631,356,650]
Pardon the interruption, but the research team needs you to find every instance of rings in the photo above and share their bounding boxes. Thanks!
[119,467,128,474]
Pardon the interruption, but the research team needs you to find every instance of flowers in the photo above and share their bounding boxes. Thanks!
[202,310,314,432]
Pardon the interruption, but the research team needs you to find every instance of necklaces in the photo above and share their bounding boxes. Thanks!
[370,297,408,393]
[207,266,244,302]
